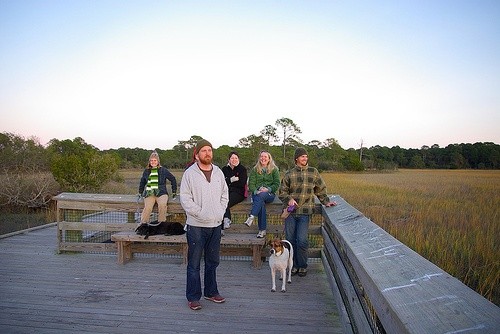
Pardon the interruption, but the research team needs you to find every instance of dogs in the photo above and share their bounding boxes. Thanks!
[267,239,294,293]
[135,220,186,239]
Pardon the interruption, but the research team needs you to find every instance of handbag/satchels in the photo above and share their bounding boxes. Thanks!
[238,164,249,198]
[280,205,292,220]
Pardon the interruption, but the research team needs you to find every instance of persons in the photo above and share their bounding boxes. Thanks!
[279,148,337,277]
[139,153,176,224]
[221,152,247,236]
[244,151,280,238]
[184,148,199,172]
[180,139,229,310]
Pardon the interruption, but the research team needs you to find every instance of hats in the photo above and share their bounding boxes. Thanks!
[186,144,197,166]
[295,148,308,159]
[196,139,212,154]
[148,150,160,169]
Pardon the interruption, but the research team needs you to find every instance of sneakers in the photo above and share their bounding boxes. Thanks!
[204,294,226,303]
[257,230,266,238]
[298,268,307,277]
[244,218,254,227]
[288,267,297,275]
[221,231,224,237]
[187,301,202,310]
[224,218,231,229]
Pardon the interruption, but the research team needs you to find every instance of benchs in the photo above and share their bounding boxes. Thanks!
[111,232,267,270]
